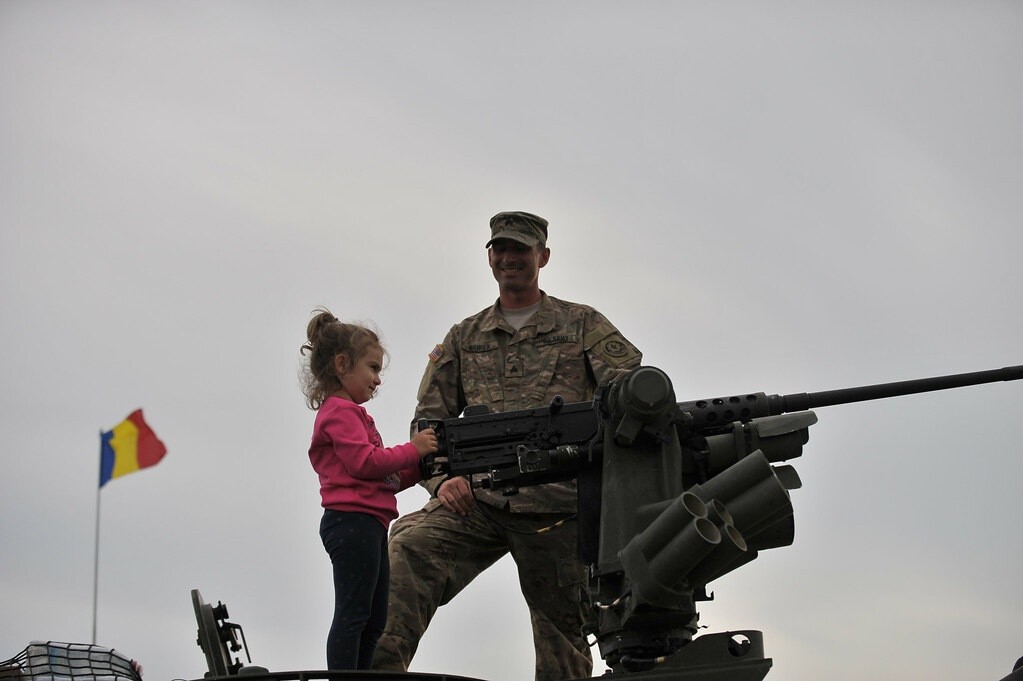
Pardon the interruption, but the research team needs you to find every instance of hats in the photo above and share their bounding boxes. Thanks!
[486,210,548,249]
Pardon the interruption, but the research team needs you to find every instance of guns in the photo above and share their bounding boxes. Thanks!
[419,365,1022,492]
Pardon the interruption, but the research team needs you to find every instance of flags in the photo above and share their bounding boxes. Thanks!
[98,407,168,491]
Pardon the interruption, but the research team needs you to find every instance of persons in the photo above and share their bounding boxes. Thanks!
[294,303,440,681]
[362,208,646,681]
